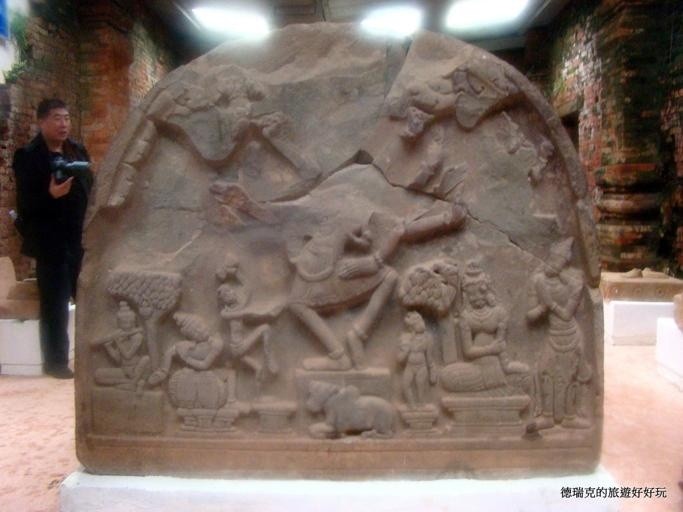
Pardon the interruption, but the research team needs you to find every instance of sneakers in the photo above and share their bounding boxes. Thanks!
[46,366,73,378]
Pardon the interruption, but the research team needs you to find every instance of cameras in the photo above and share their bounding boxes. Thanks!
[54,156,90,185]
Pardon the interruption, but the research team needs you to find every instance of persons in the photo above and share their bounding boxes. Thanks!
[395,303,438,411]
[524,234,592,436]
[93,298,153,399]
[437,261,531,393]
[212,252,285,379]
[206,176,405,375]
[146,309,252,418]
[13,97,95,378]
